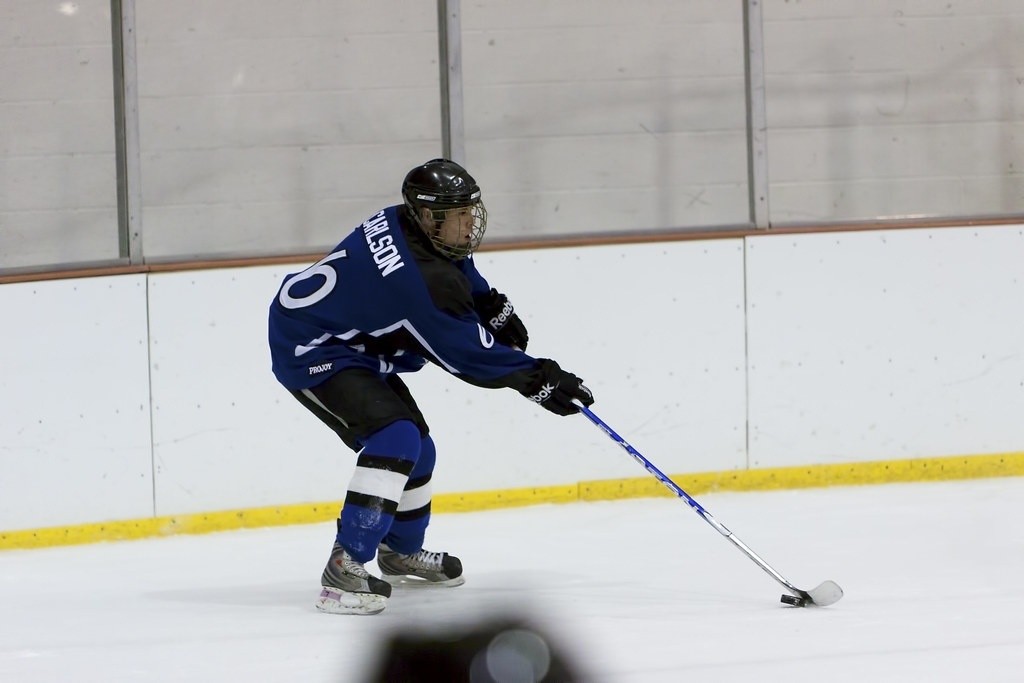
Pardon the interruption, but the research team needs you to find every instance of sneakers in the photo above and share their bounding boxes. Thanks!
[316,518,391,615]
[377,543,465,587]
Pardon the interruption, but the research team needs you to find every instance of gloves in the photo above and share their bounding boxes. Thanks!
[518,358,594,416]
[476,288,529,352]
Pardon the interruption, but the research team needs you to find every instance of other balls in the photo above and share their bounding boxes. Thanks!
[781,593,806,608]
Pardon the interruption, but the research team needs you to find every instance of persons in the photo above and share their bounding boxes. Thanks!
[267,156,596,615]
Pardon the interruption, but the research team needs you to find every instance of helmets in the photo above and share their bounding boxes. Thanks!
[402,158,481,210]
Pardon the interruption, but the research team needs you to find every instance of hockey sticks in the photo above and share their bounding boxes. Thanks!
[570,396,844,606]
